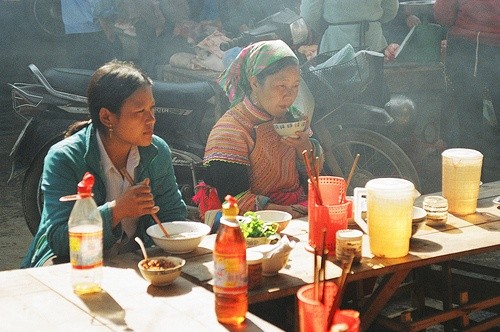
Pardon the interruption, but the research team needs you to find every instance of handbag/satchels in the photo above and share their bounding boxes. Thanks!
[307,43,370,98]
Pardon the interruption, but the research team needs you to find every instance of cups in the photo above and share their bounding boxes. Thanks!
[336,229,363,261]
[423,196,448,227]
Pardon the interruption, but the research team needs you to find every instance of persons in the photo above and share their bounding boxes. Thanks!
[20,60,188,270]
[60,1,422,106]
[202,40,325,220]
[431,0,500,183]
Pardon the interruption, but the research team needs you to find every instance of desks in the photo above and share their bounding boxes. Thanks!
[1,180,500,332]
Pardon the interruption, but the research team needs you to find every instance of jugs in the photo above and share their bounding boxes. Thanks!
[441,148,483,215]
[353,178,421,259]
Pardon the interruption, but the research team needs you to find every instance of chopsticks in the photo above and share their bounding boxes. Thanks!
[313,229,355,329]
[121,168,168,237]
[302,149,359,205]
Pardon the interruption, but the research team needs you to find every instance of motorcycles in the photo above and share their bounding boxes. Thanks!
[6,7,421,239]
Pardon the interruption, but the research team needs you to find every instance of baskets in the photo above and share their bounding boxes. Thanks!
[300,45,374,107]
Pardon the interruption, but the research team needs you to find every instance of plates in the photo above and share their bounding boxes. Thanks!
[146,220,212,254]
[492,196,500,205]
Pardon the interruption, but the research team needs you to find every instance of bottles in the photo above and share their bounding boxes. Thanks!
[213,195,248,326]
[246,251,263,290]
[68,172,103,296]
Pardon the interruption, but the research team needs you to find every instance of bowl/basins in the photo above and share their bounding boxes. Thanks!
[255,210,292,233]
[345,196,367,226]
[253,244,292,276]
[412,206,427,235]
[245,237,271,248]
[137,256,186,287]
[480,181,482,187]
[273,120,306,139]
